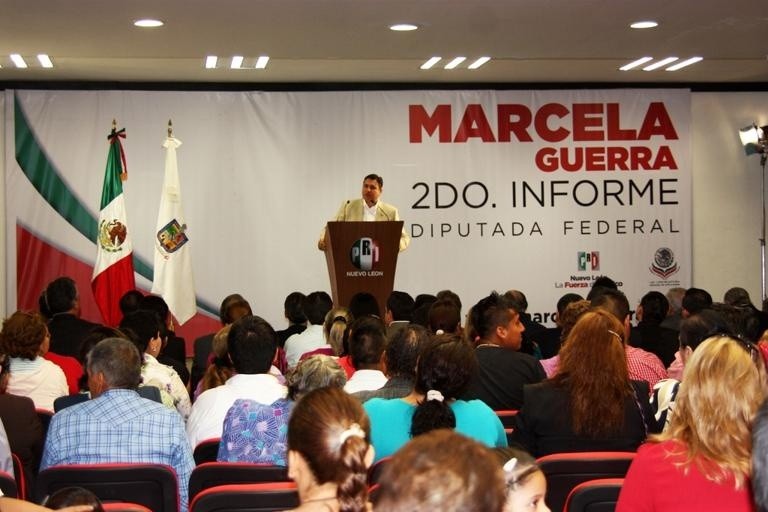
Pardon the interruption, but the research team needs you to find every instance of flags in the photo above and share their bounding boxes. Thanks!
[152,116,198,330]
[92,120,134,327]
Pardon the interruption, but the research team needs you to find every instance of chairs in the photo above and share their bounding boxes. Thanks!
[536,451,636,512]
[189,463,295,502]
[191,482,301,511]
[192,437,221,466]
[30,462,180,512]
[563,478,625,512]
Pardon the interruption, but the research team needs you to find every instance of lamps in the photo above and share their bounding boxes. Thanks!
[737,123,768,166]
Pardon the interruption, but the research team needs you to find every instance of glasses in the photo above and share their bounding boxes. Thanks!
[722,332,759,358]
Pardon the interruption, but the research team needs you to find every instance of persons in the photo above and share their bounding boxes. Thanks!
[669,286,726,345]
[470,292,548,429]
[216,353,348,467]
[335,428,507,512]
[287,387,375,512]
[111,288,193,419]
[348,325,432,404]
[485,445,549,512]
[408,294,437,329]
[0,311,70,416]
[346,292,387,335]
[500,289,548,361]
[190,316,289,451]
[627,291,681,371]
[299,307,354,354]
[283,291,332,370]
[437,291,461,311]
[427,300,461,337]
[198,290,284,398]
[362,335,507,463]
[647,307,736,438]
[317,173,411,251]
[539,291,589,360]
[37,276,124,393]
[512,311,649,511]
[538,289,666,395]
[0,350,45,502]
[40,339,196,512]
[384,291,415,338]
[273,292,307,349]
[335,314,387,379]
[724,288,768,336]
[616,336,766,512]
